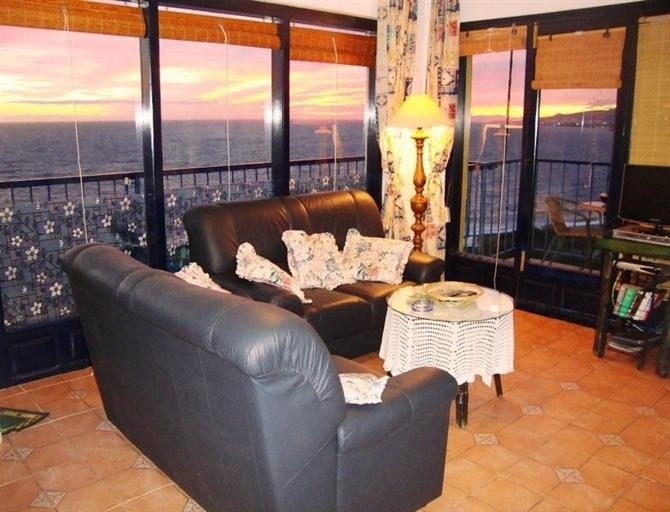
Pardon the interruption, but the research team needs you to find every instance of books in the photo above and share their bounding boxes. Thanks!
[612,283,667,321]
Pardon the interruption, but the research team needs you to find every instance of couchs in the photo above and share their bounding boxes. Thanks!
[183,189,444,360]
[60,241,460,512]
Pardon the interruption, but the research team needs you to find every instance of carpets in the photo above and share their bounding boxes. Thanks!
[0,406,50,435]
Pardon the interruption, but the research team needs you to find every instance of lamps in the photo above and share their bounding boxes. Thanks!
[386,94,455,252]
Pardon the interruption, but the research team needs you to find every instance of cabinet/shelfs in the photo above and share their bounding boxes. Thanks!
[593,237,669,379]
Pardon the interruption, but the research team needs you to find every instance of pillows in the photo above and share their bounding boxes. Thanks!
[338,373,390,405]
[235,229,415,304]
[173,262,232,295]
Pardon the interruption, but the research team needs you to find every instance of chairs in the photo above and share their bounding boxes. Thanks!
[541,196,598,271]
[595,256,667,371]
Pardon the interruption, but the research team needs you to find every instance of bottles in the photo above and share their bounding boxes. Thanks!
[411,291,433,312]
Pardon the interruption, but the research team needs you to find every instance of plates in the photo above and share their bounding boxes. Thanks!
[422,282,485,304]
